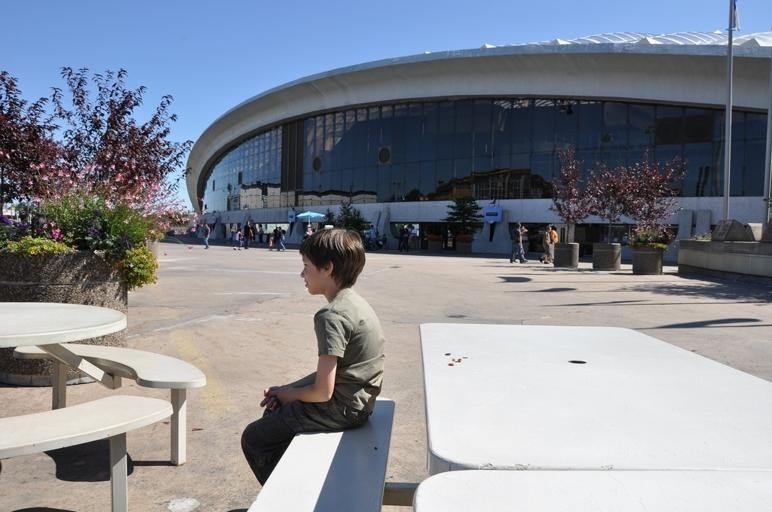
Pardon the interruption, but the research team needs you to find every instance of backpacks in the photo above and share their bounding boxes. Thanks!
[281,233,285,241]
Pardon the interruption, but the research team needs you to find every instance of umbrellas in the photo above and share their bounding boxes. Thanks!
[295,211,326,224]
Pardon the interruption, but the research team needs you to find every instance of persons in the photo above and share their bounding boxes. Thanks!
[201,224,211,248]
[242,227,387,487]
[398,223,417,252]
[230,220,288,251]
[367,224,387,251]
[539,223,559,264]
[509,223,529,263]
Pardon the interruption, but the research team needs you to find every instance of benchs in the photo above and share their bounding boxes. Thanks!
[0,346,207,512]
[243,396,398,511]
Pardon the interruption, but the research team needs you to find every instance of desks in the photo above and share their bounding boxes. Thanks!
[0,300,122,417]
[414,468,770,511]
[418,322,771,475]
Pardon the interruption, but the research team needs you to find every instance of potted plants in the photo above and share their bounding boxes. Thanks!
[552,143,690,274]
[428,234,443,252]
[455,235,474,255]
